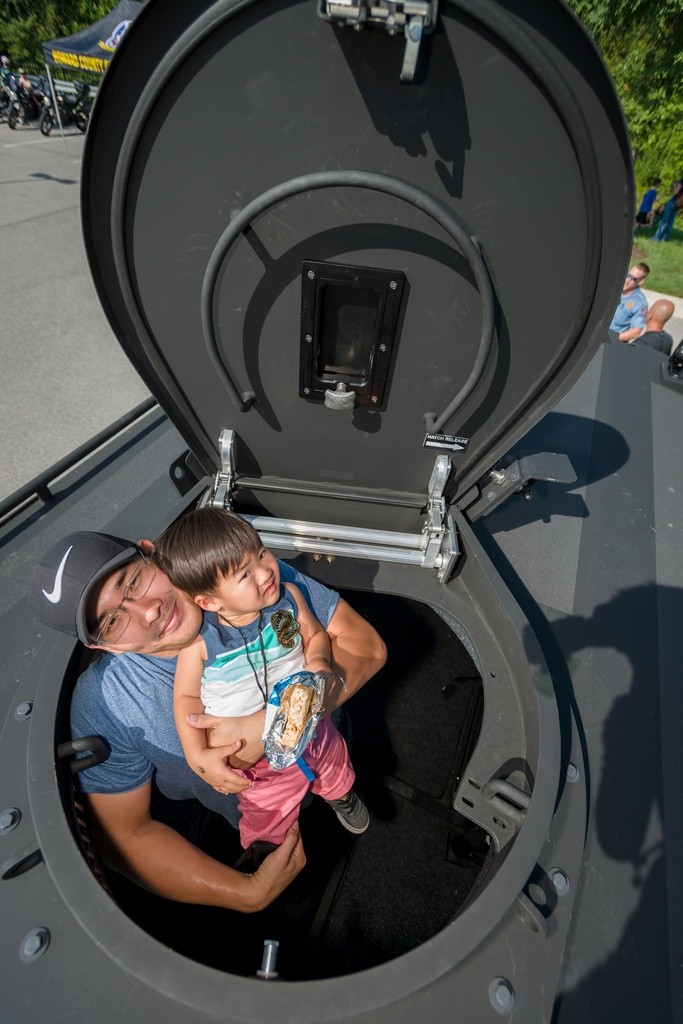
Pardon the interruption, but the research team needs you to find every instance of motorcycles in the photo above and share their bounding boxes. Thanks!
[1,74,91,137]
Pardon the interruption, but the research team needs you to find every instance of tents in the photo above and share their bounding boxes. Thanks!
[40,0,150,157]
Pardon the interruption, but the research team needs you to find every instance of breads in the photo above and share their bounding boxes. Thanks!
[280,684,316,748]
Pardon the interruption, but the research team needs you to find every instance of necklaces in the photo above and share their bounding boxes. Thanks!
[218,613,268,709]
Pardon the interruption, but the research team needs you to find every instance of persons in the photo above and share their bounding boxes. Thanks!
[152,506,370,870]
[630,298,675,356]
[27,531,386,912]
[0,56,41,125]
[634,178,683,242]
[609,262,651,341]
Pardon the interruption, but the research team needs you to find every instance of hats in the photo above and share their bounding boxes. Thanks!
[28,530,142,646]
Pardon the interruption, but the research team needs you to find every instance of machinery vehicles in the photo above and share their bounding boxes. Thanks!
[0,0,683,1024]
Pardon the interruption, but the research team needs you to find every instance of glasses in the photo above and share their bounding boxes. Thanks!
[626,274,639,281]
[95,550,155,647]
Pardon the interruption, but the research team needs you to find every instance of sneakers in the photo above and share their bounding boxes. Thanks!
[327,787,370,834]
